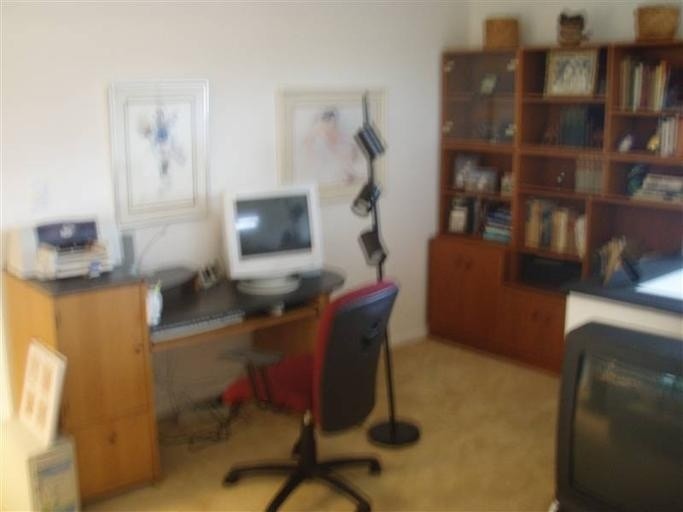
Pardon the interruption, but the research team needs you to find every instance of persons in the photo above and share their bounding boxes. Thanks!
[549,56,591,96]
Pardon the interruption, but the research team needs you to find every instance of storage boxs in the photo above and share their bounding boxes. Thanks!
[2,416,81,511]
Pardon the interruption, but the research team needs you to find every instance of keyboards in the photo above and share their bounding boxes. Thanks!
[148,310,245,344]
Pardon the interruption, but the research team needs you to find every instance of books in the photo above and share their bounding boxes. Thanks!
[477,197,585,259]
[39,245,112,281]
[573,56,682,203]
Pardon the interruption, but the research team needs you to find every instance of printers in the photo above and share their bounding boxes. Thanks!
[6,215,123,282]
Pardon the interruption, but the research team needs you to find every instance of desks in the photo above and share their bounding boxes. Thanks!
[152,267,340,412]
[563,255,683,341]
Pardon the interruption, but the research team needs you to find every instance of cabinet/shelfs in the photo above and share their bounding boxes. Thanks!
[429,47,683,376]
[3,266,157,504]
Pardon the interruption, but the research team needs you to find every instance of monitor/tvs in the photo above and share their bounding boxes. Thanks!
[223,184,324,296]
[556,322,683,512]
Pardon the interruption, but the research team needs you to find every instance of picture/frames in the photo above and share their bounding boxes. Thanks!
[108,78,212,230]
[274,83,388,198]
[542,47,601,98]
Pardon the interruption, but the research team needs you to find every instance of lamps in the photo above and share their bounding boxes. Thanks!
[351,91,421,447]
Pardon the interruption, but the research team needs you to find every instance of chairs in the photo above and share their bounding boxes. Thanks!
[219,276,400,511]
